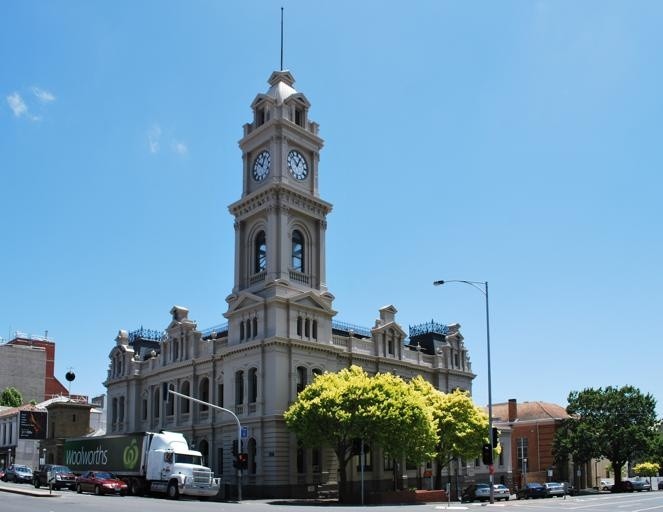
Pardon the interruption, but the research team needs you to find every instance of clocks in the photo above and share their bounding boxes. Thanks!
[251,150,273,182]
[287,150,309,180]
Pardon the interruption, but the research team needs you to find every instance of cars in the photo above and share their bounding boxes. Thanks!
[0,463,128,496]
[658,481,663,491]
[599,479,651,493]
[460,481,511,503]
[515,482,580,500]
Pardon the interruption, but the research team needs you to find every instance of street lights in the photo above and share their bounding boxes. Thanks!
[522,457,529,486]
[547,468,555,482]
[432,277,496,505]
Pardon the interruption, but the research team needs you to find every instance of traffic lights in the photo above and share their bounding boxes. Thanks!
[482,428,498,465]
[232,439,249,470]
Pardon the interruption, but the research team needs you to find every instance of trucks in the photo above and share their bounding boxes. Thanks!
[63,430,222,501]
